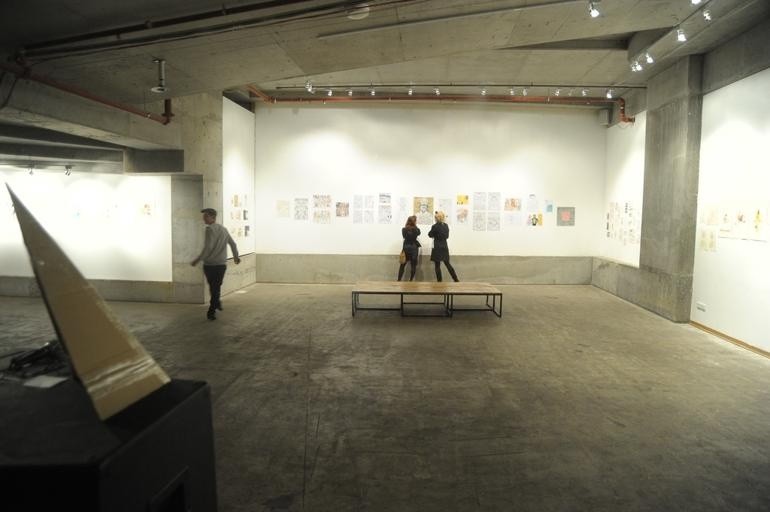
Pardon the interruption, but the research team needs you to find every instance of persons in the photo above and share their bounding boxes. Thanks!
[396,216,421,281]
[427,211,460,283]
[414,200,433,224]
[190,208,240,321]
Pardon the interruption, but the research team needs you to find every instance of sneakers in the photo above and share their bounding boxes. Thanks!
[207,314,217,321]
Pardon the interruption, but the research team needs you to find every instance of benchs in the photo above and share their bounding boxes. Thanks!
[352,280,502,317]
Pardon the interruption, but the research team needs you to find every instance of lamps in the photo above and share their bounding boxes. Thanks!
[630,52,654,72]
[275,80,647,99]
[675,25,687,42]
[702,5,712,21]
[587,0,602,18]
[344,1,369,21]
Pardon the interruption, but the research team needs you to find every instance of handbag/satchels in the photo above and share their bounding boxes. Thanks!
[430,247,435,261]
[398,248,407,264]
